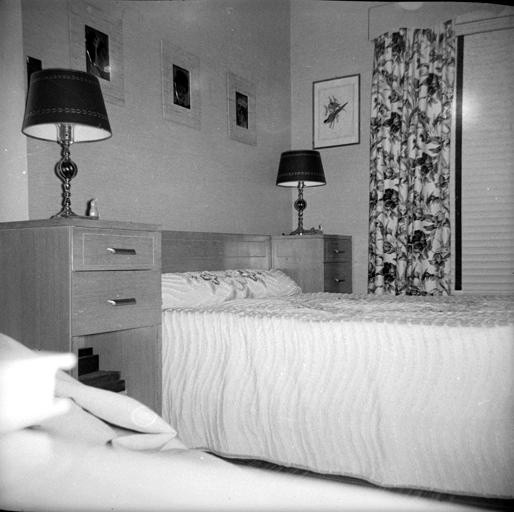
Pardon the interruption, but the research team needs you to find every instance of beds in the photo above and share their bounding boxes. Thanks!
[162,230,514,512]
[0,326,475,512]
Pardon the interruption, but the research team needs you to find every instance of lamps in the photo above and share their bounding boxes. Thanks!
[274,148,326,237]
[20,69,114,221]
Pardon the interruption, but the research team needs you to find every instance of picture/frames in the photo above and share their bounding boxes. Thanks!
[156,38,205,134]
[310,74,361,150]
[64,0,128,112]
[223,69,259,147]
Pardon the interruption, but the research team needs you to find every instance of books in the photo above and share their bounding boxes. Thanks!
[77,345,128,398]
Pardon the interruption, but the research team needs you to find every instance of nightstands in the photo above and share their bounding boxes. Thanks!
[0,216,164,421]
[269,231,354,295]
